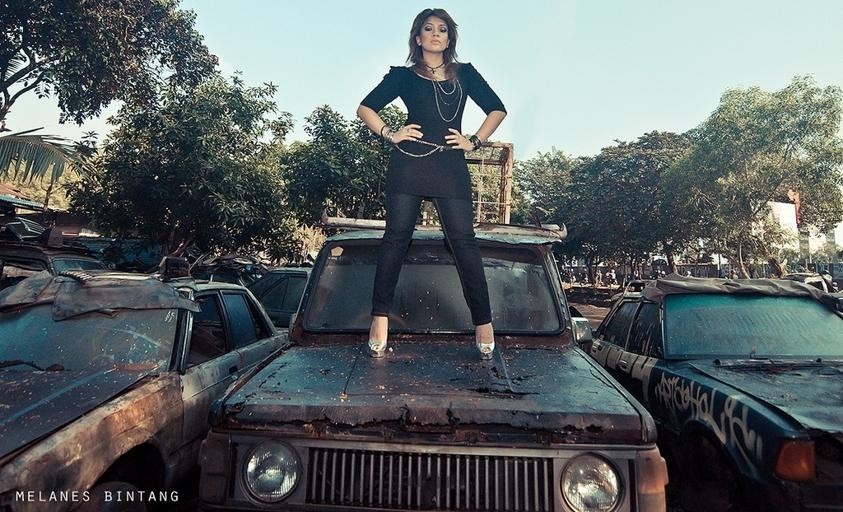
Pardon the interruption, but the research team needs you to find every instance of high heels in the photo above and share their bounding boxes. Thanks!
[476,342,495,360]
[368,340,387,358]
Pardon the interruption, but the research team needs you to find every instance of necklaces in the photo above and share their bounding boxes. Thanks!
[424,60,464,123]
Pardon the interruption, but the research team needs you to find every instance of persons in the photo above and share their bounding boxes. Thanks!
[356,9,506,358]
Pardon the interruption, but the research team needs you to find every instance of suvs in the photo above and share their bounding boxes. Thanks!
[184,214,678,512]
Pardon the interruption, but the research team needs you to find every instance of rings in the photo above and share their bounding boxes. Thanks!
[406,128,410,132]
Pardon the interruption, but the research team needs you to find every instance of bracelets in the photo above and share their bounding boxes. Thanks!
[469,135,482,149]
[381,125,394,143]
